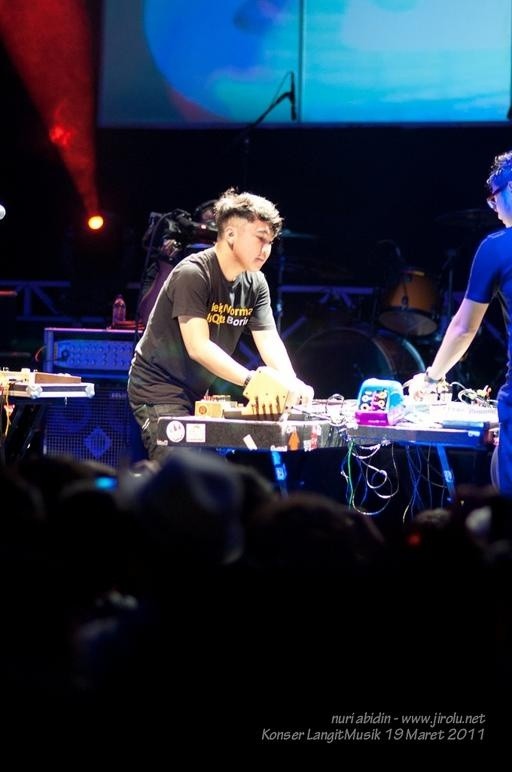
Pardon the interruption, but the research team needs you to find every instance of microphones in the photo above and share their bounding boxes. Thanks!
[291,73,297,120]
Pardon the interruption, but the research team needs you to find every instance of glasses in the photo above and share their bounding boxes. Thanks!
[486,182,509,211]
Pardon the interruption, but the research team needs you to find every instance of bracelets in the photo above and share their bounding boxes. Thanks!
[243,368,257,391]
[424,366,445,385]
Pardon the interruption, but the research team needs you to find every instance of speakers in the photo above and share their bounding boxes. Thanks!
[42,373,149,471]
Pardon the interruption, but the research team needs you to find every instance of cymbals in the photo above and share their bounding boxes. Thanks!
[278,230,312,237]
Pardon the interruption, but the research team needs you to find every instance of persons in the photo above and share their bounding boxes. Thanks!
[403,147,512,499]
[126,185,316,465]
[0,441,511,772]
[137,196,221,329]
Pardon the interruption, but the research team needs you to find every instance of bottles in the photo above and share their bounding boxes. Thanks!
[112,294,126,322]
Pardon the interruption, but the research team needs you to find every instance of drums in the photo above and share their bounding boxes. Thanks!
[291,324,425,398]
[377,273,441,336]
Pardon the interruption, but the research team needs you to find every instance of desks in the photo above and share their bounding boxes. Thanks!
[0,382,95,467]
[157,398,499,503]
